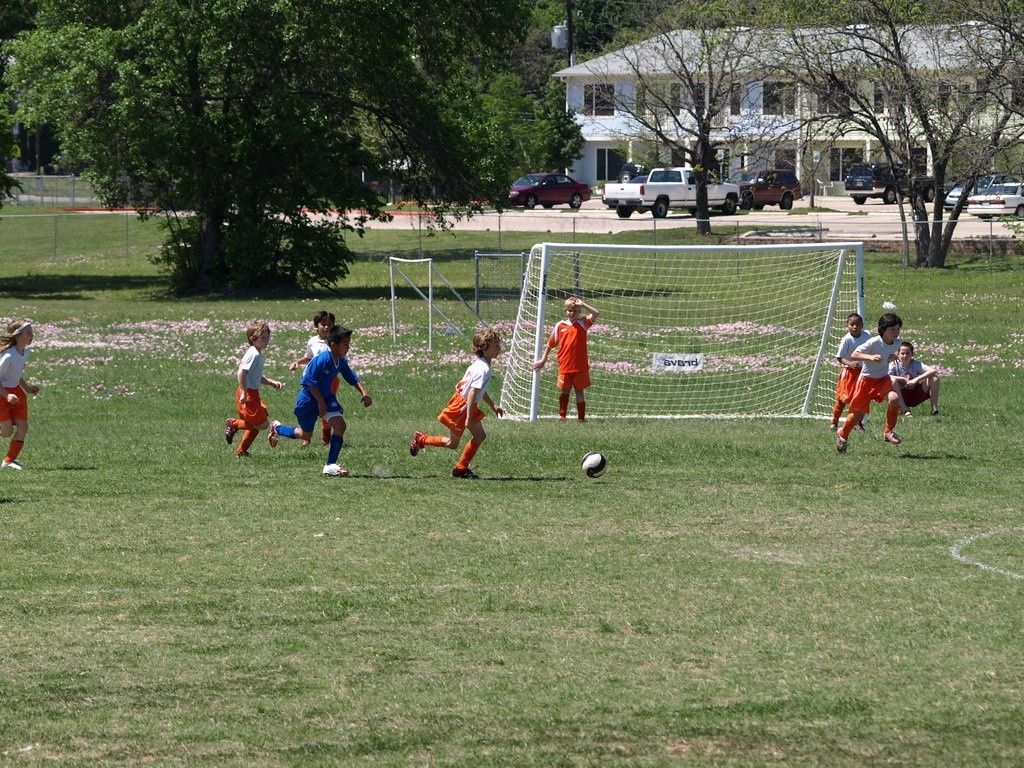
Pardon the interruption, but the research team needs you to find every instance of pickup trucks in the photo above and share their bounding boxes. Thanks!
[601,166,740,218]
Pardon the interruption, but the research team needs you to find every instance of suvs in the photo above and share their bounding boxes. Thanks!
[844,162,936,205]
[724,168,804,210]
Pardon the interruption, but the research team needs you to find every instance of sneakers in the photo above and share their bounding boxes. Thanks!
[453,465,479,479]
[2,459,25,471]
[836,427,846,454]
[323,463,348,476]
[410,431,424,456]
[224,418,238,444]
[234,449,251,457]
[884,430,901,444]
[268,420,282,448]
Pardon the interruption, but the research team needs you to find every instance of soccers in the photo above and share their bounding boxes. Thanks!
[581,451,607,478]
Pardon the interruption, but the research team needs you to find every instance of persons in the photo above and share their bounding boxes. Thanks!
[533,296,599,421]
[225,321,282,457]
[289,311,339,444]
[888,341,939,418]
[0,319,39,471]
[836,313,901,454]
[409,329,503,480]
[268,325,372,476]
[830,313,872,431]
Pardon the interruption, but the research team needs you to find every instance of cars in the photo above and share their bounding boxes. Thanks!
[967,182,1024,222]
[615,159,676,186]
[942,175,1023,212]
[504,173,590,209]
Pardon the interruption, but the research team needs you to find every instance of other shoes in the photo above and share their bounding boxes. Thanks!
[830,423,837,432]
[931,411,940,416]
[904,411,914,418]
[302,438,310,446]
[856,422,864,433]
[322,427,331,445]
